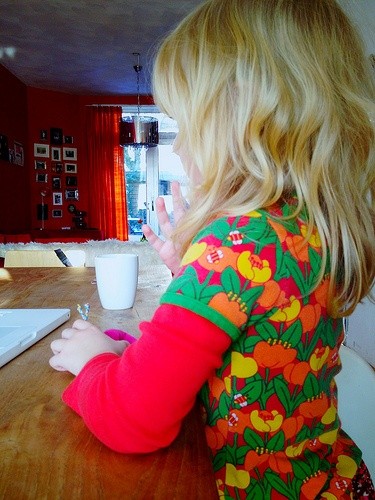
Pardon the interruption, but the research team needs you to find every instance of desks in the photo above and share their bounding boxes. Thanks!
[0,266,220,500]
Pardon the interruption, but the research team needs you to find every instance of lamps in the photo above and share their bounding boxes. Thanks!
[119,54,159,149]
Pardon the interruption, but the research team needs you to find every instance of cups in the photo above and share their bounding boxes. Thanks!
[0,243,7,268]
[94,252,139,311]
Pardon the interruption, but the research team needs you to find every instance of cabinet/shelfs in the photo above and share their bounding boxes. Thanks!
[0,226,101,244]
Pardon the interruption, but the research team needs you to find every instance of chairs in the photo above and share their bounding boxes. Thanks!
[4,250,86,268]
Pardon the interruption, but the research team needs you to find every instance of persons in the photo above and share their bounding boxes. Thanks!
[49,0,375,500]
